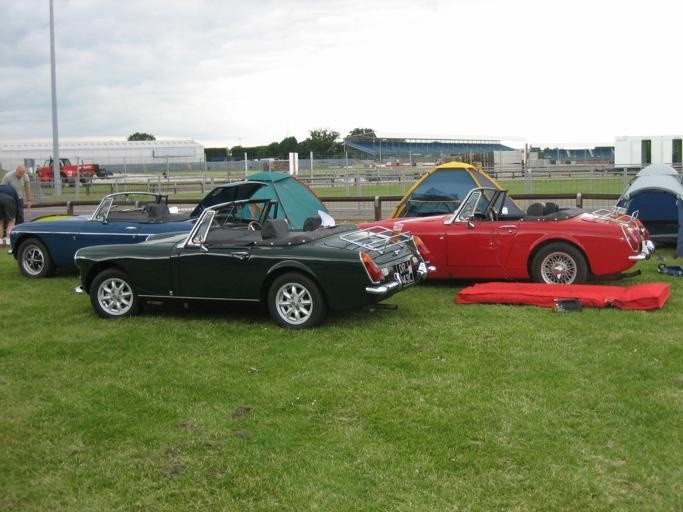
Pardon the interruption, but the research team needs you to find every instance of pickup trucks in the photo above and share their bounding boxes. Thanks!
[35,156,98,186]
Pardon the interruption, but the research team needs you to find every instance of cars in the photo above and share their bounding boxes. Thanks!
[412,170,426,180]
[590,166,608,173]
[334,166,402,184]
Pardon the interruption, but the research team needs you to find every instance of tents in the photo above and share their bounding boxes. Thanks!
[190,171,334,231]
[615,163,683,259]
[388,161,526,219]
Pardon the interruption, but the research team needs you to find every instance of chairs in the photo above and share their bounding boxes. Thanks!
[303,216,321,232]
[261,220,288,240]
[545,203,559,216]
[150,205,170,218]
[527,203,544,216]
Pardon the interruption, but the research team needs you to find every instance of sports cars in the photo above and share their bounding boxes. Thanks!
[7,180,279,278]
[353,186,655,286]
[73,198,436,329]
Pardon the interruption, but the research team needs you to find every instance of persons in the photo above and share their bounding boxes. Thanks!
[0,164,32,236]
[0,185,19,254]
